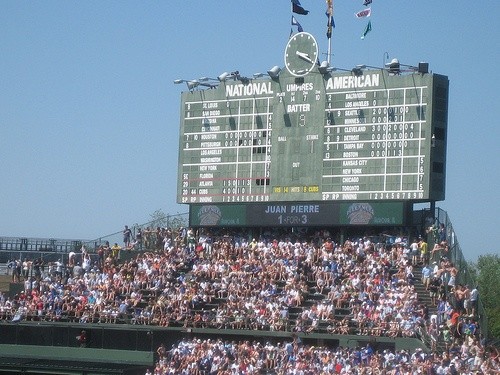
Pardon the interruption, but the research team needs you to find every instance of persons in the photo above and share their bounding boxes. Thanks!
[0,213,499,375]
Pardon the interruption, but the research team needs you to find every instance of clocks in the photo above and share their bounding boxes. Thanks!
[284,32,318,77]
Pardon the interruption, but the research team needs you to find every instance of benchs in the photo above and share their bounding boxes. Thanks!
[0,229,405,336]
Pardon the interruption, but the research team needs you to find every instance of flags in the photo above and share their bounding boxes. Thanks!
[291,0,301,5]
[292,5,309,15]
[356,9,370,18]
[292,16,303,32]
[361,20,371,41]
[363,0,372,6]
[326,0,335,28]
